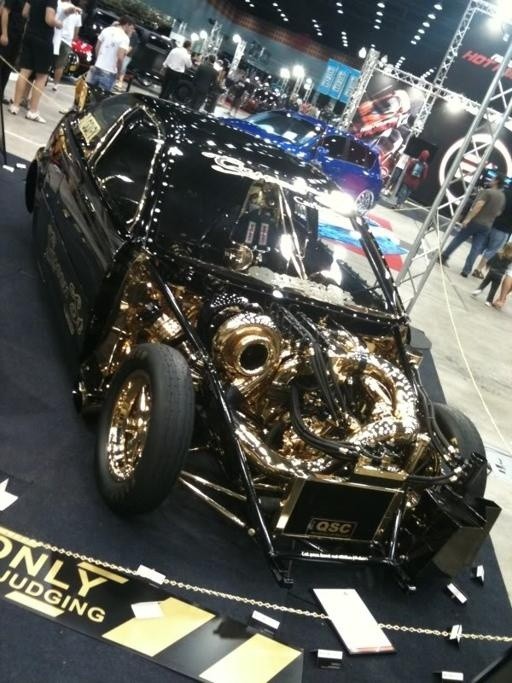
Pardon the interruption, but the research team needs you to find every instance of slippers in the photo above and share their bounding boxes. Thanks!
[411,158,427,179]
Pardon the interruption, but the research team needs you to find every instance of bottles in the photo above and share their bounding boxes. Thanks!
[420,150,430,160]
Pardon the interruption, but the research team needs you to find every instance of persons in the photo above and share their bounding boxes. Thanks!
[46,0,83,92]
[471,179,511,280]
[8,0,64,123]
[392,149,430,209]
[84,14,136,93]
[471,241,511,307]
[1,0,27,106]
[435,173,507,278]
[22,0,83,109]
[158,37,336,123]
[491,262,511,311]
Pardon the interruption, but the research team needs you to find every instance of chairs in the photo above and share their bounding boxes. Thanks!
[471,288,505,309]
[2,96,24,115]
[52,85,58,92]
[58,108,70,115]
[437,249,449,264]
[460,263,483,279]
[25,110,47,124]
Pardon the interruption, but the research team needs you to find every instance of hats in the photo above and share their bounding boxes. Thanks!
[350,89,409,183]
[19,89,501,585]
[43,9,204,107]
[212,108,383,216]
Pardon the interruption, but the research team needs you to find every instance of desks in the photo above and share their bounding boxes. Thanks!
[187,19,314,106]
